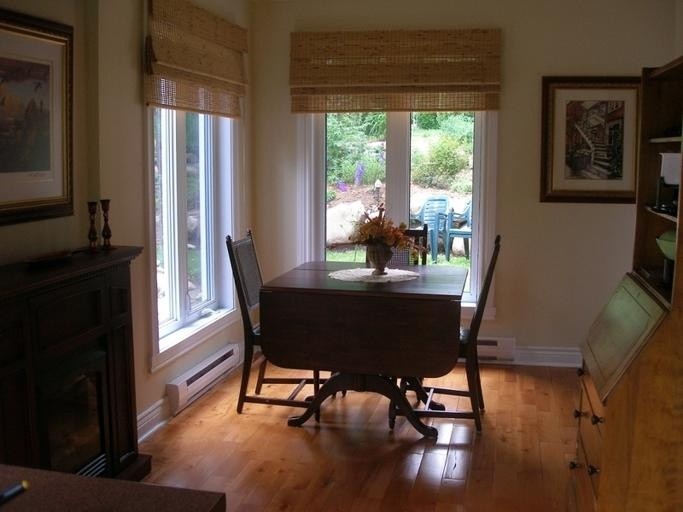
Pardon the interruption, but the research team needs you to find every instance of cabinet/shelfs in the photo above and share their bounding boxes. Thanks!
[2,244,153,483]
[566,54,683,509]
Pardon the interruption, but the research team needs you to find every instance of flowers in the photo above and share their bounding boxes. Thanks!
[348,203,431,261]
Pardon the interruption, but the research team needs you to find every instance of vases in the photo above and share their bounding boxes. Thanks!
[366,243,388,275]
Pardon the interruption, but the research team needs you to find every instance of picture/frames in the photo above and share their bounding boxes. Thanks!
[0,8,75,225]
[539,76,642,206]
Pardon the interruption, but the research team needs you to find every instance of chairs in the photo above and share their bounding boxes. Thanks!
[385,232,504,432]
[225,228,347,424]
[409,196,449,226]
[342,223,426,402]
[426,212,447,266]
[444,199,472,262]
